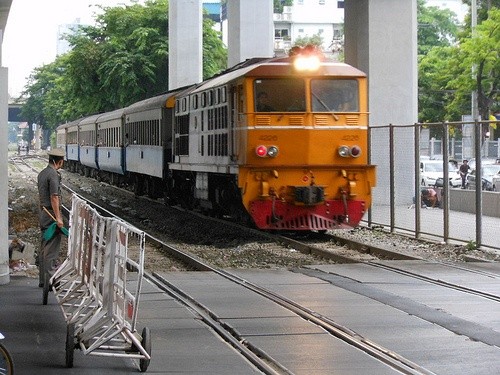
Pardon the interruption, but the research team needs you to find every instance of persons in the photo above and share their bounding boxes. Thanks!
[18,146,20,154]
[38,147,66,291]
[27,145,29,155]
[459,160,470,187]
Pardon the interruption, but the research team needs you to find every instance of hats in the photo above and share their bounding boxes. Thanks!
[463,160,468,163]
[46,147,66,156]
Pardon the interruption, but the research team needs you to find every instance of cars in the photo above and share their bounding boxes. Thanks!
[464,163,500,192]
[419,159,463,189]
[467,157,499,169]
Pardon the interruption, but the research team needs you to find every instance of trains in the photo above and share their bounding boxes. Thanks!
[55,42,378,240]
